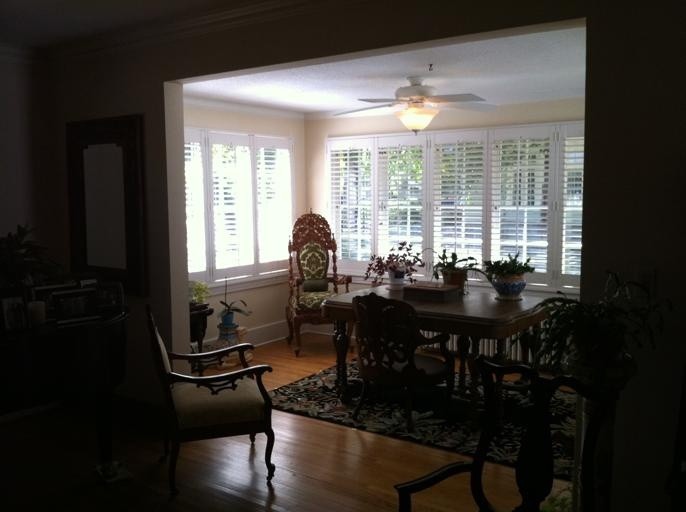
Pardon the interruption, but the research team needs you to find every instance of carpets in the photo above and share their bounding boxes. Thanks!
[266,357,586,480]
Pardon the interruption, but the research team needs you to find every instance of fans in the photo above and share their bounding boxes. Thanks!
[334,76,501,119]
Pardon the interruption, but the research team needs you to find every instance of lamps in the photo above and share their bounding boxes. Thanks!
[395,102,440,137]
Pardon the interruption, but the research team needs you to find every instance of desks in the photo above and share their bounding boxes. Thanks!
[320,285,552,416]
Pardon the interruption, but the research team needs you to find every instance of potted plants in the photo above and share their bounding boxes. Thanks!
[364,242,536,303]
[188,282,252,344]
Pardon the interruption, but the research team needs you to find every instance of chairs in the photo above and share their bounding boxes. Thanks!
[286,212,354,358]
[143,305,276,494]
